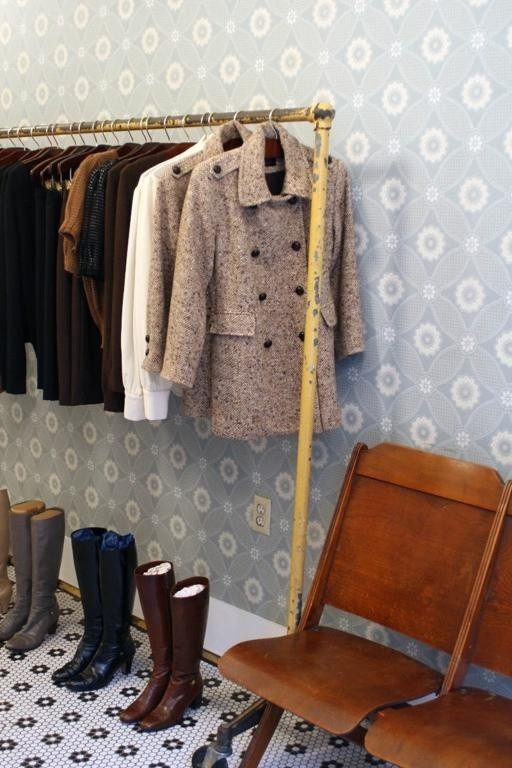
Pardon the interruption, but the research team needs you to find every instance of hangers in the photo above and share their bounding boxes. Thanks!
[2,109,311,191]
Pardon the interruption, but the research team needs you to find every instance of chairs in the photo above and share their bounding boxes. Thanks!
[218,442,506,768]
[365,474,512,768]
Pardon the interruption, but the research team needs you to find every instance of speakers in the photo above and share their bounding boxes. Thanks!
[51,527,210,731]
[1,489,65,651]
